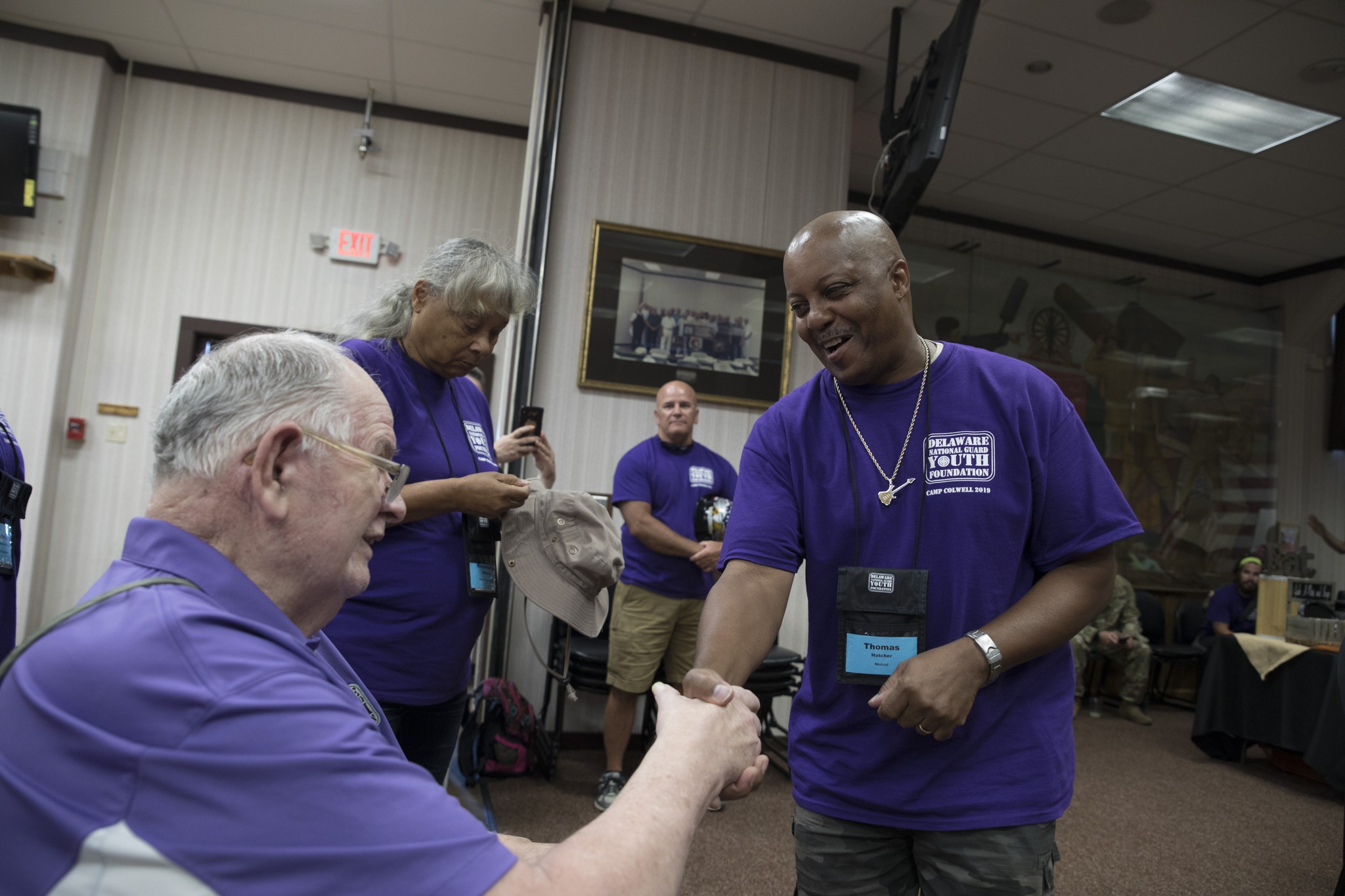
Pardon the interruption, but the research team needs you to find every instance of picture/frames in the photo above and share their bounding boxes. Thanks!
[577,218,794,409]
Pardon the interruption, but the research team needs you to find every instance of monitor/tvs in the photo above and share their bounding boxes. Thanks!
[881,0,980,234]
[0,101,43,217]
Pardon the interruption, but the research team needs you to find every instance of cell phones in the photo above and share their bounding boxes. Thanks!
[518,406,543,449]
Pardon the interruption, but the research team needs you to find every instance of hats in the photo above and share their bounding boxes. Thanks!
[499,475,624,638]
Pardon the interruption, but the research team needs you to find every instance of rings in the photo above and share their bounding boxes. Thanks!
[918,723,934,735]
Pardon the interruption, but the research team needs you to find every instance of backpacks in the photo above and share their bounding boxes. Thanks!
[457,677,552,784]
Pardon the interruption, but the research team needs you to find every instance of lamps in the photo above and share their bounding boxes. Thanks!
[310,229,401,265]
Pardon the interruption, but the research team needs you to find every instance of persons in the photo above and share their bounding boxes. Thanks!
[461,365,559,490]
[303,235,532,787]
[1207,554,1263,640]
[1069,572,1152,725]
[1,328,762,896]
[629,300,754,362]
[593,380,738,813]
[681,209,1144,896]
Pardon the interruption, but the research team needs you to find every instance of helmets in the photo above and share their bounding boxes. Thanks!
[707,495,735,541]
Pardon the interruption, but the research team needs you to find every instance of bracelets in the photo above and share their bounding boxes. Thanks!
[963,629,1003,686]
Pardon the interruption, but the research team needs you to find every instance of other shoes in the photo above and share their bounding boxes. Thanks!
[594,770,621,811]
[1117,697,1153,724]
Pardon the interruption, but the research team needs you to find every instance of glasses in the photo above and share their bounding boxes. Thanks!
[243,429,411,503]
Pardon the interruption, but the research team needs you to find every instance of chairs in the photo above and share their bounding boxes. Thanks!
[540,615,805,785]
[1087,585,1210,712]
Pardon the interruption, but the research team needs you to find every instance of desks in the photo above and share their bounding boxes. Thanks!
[1190,630,1345,807]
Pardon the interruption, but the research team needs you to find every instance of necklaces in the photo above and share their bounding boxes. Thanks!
[832,333,931,507]
